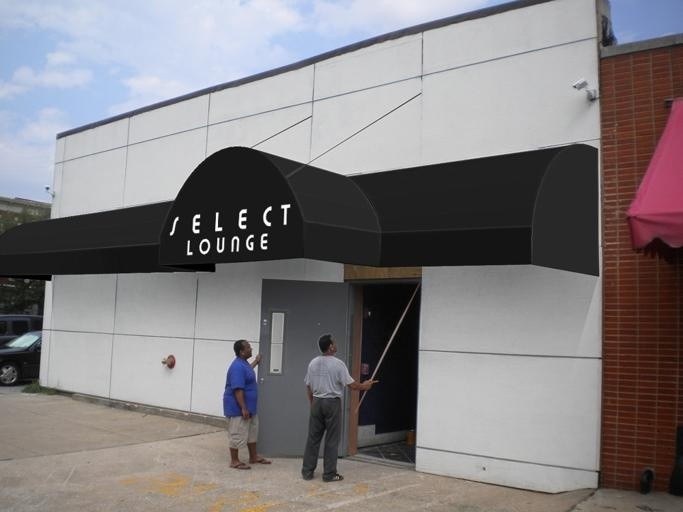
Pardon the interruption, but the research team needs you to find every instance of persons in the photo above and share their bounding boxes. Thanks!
[223,340,273,469]
[300,333,380,482]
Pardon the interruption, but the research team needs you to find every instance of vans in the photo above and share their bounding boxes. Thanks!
[0,314,42,347]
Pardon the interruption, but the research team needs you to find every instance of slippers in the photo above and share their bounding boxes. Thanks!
[230,463,251,469]
[249,459,271,464]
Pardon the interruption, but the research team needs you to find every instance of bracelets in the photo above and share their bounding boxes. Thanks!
[254,359,258,364]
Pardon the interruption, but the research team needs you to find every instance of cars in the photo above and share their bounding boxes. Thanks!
[0,329,42,387]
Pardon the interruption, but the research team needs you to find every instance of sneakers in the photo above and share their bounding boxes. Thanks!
[332,474,343,481]
